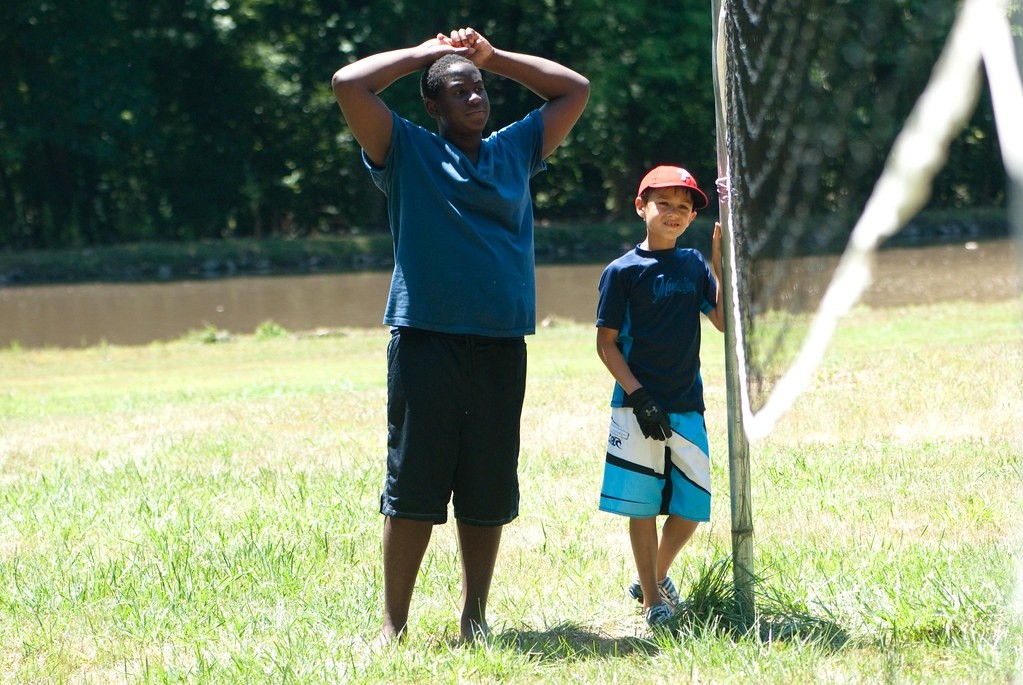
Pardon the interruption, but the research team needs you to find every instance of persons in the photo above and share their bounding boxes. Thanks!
[596,165,724,631]
[331,27,591,655]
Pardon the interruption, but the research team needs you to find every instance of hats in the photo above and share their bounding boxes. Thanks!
[638,164,709,210]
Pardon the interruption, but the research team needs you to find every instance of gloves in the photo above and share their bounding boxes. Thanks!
[630,388,672,442]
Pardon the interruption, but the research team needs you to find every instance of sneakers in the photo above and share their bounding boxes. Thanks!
[629,577,681,612]
[643,604,678,639]
